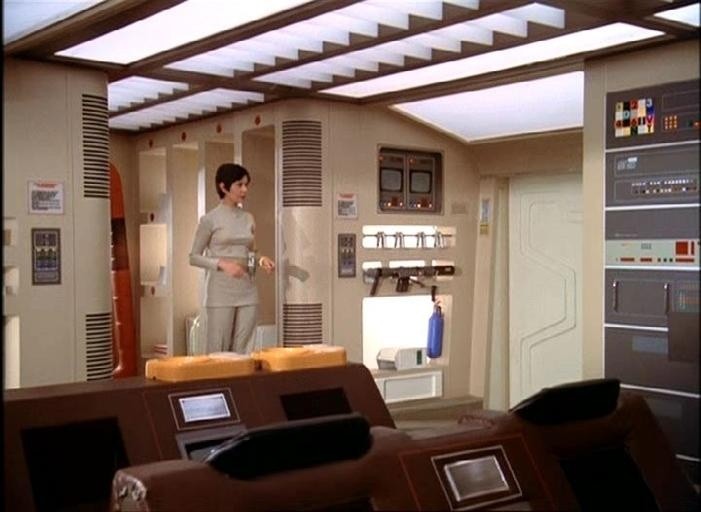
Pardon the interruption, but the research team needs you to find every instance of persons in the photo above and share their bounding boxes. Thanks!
[187,162,277,353]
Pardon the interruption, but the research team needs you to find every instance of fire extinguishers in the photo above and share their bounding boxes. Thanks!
[427,301,443,358]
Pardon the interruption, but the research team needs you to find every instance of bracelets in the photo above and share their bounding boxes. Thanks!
[258,255,268,268]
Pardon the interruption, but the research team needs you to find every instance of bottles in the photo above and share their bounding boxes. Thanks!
[427,306,445,359]
